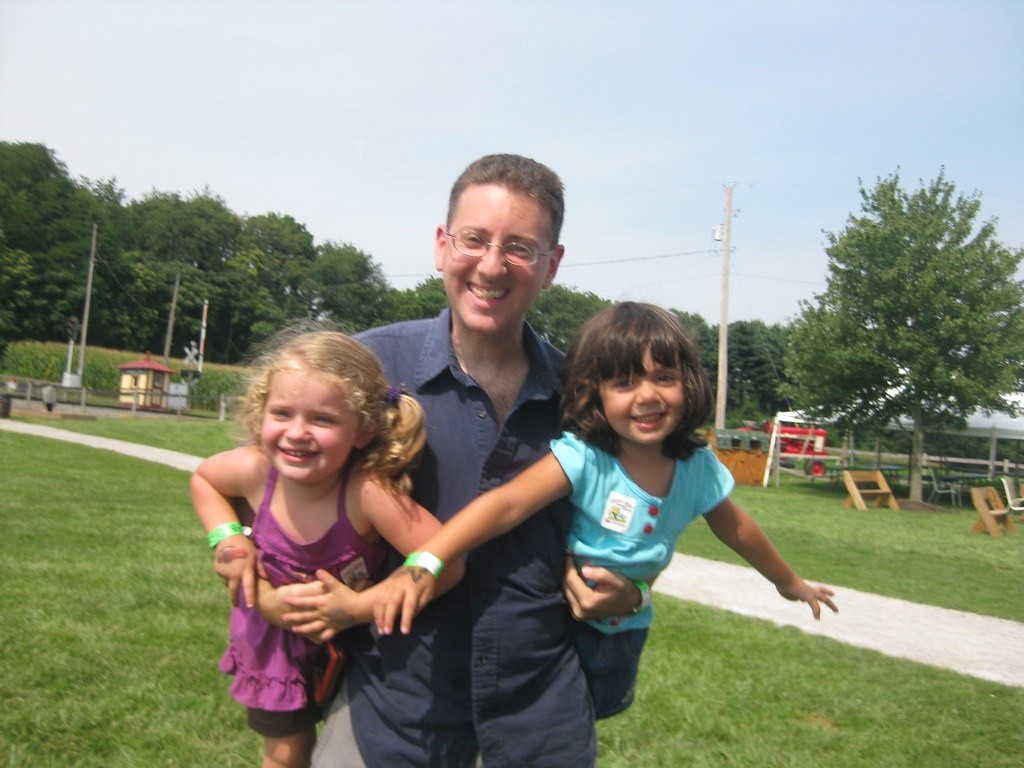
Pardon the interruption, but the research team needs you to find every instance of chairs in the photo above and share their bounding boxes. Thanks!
[928,467,961,507]
[841,469,899,512]
[970,487,1016,536]
[999,476,1024,513]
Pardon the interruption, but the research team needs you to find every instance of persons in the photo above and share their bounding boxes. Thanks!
[372,303,839,723]
[251,154,655,768]
[191,331,466,768]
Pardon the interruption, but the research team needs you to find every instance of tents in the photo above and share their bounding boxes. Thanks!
[763,373,1024,489]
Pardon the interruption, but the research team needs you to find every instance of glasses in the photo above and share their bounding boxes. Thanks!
[443,231,556,267]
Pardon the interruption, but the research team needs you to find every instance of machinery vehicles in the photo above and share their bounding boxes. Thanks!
[736,407,830,480]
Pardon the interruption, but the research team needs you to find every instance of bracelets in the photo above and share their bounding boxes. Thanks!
[634,581,650,615]
[207,522,245,549]
[403,552,445,579]
